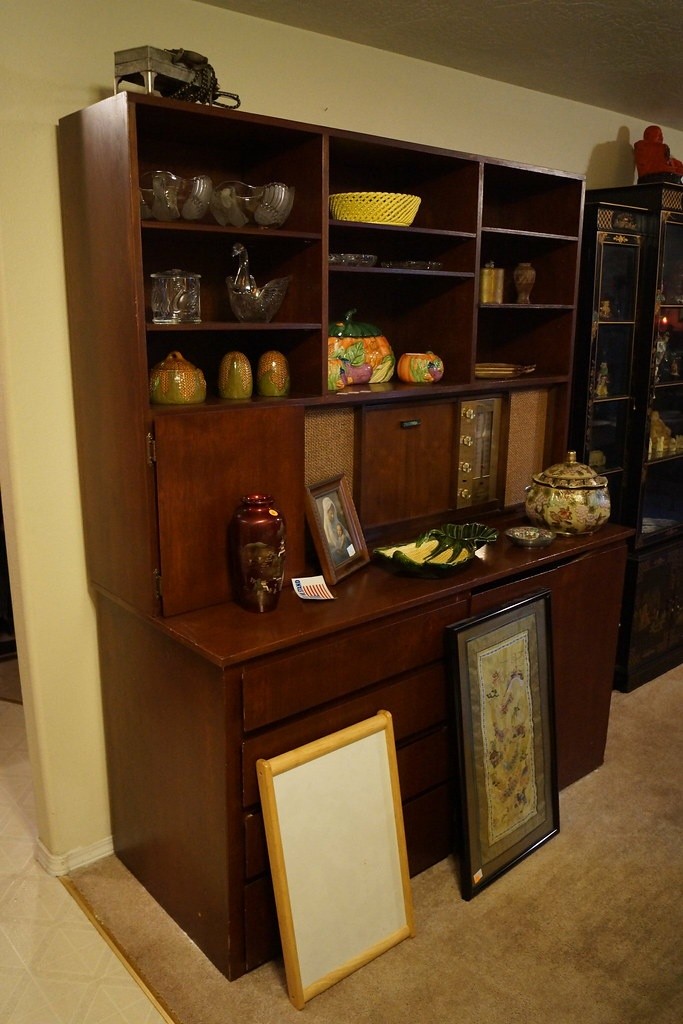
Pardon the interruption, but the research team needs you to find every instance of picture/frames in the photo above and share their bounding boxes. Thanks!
[305,473,372,585]
[256,708,415,1009]
[443,585,561,900]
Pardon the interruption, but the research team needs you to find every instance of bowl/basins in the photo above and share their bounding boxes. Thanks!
[138,170,213,223]
[340,254,377,267]
[504,526,556,550]
[211,180,295,230]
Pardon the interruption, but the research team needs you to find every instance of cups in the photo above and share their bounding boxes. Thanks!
[150,274,183,325]
[177,274,203,325]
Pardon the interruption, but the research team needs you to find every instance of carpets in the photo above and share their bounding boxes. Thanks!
[0,630,22,706]
[57,658,683,1024]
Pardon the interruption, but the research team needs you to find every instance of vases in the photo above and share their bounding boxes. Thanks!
[231,492,284,613]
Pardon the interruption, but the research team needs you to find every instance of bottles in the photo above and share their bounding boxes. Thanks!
[235,494,286,614]
[514,261,536,304]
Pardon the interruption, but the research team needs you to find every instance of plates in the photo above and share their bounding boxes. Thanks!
[403,261,443,271]
[372,522,499,580]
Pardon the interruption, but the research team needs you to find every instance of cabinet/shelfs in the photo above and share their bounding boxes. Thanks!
[59,89,586,617]
[472,544,627,792]
[585,200,647,512]
[594,182,683,692]
[100,595,469,983]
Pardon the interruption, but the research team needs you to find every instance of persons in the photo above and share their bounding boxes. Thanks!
[650,410,672,450]
[634,126,683,176]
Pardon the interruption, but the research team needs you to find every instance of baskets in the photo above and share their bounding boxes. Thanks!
[329,191,421,225]
